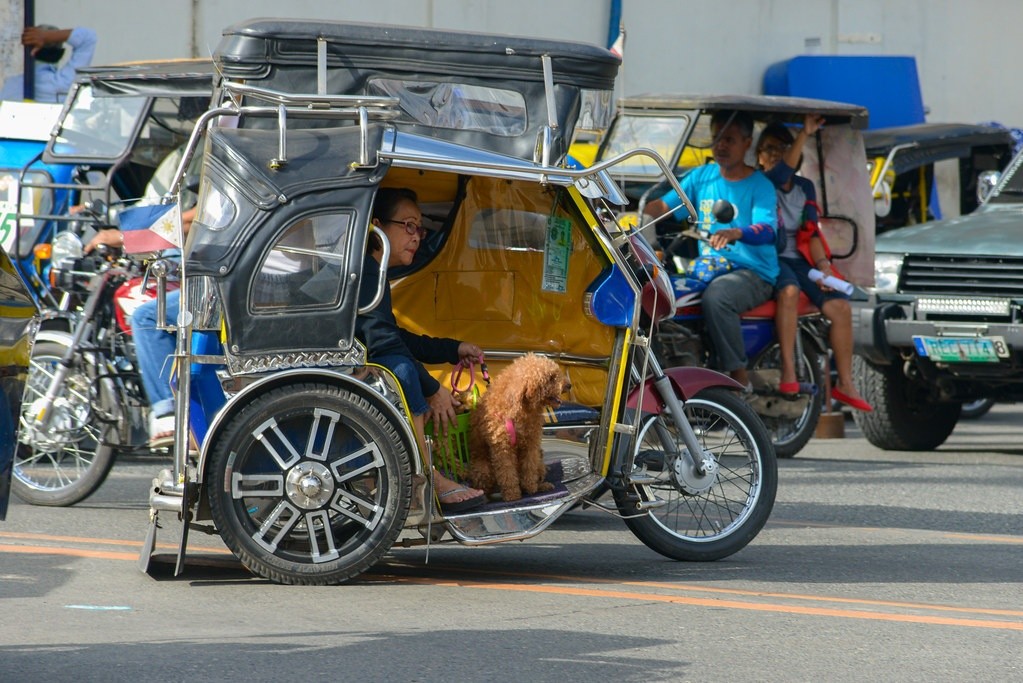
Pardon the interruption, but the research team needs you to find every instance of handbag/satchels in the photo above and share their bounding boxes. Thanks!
[686,254,731,283]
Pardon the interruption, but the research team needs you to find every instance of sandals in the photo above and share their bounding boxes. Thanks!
[147,429,175,448]
[438,484,489,512]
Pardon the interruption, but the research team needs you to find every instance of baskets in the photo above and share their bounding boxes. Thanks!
[425,383,480,484]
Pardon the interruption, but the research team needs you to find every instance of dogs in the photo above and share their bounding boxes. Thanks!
[424,352,572,502]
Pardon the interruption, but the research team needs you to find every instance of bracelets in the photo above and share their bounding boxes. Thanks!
[120,231,125,250]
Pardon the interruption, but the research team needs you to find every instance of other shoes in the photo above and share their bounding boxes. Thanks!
[779,377,800,395]
[832,385,872,412]
[730,380,753,401]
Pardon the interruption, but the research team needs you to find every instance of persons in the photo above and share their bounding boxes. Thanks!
[639,112,876,413]
[0,24,98,105]
[318,187,488,511]
[82,206,199,449]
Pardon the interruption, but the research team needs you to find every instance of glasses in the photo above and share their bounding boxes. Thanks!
[758,144,792,153]
[388,219,427,239]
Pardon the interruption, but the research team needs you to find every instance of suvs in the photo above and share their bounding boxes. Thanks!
[845,149,1023,451]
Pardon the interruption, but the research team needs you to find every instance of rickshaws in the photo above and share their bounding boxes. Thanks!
[0,15,1013,510]
[135,81,780,586]
